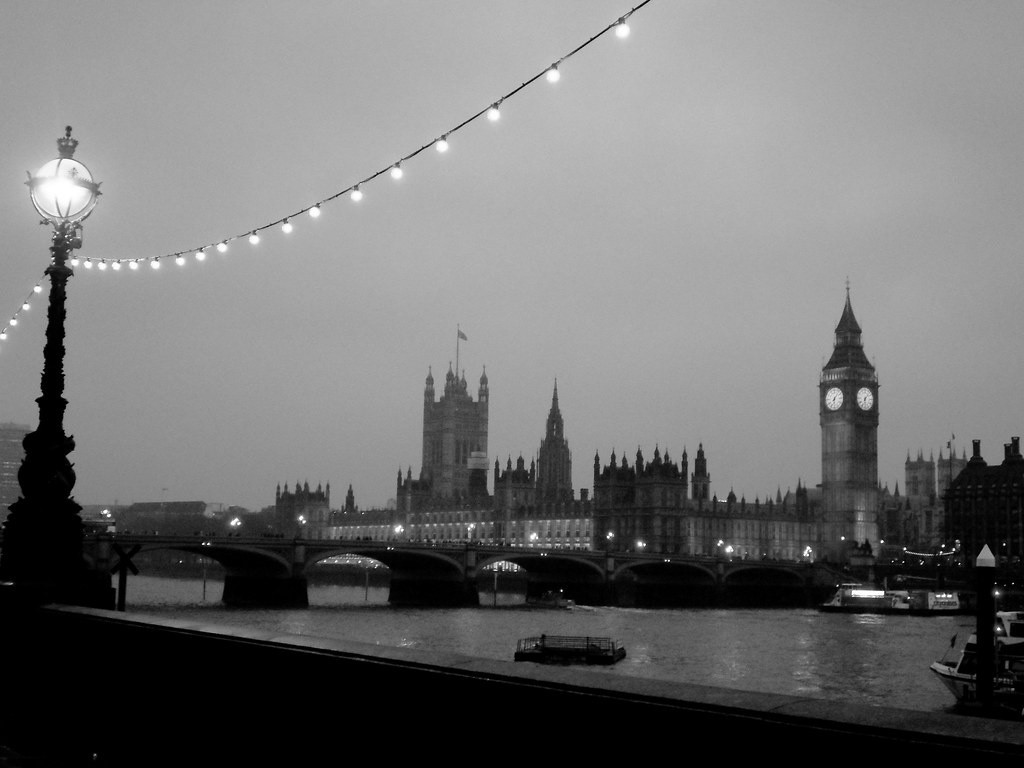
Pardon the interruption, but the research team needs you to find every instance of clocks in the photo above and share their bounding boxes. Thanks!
[824,386,843,411]
[857,386,874,411]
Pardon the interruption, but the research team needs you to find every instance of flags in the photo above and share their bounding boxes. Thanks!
[458,330,466,340]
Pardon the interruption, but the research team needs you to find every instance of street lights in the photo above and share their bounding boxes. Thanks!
[2,126,103,599]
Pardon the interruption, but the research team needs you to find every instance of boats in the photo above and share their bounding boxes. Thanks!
[930,611,1024,716]
[818,582,971,616]
[513,633,627,666]
